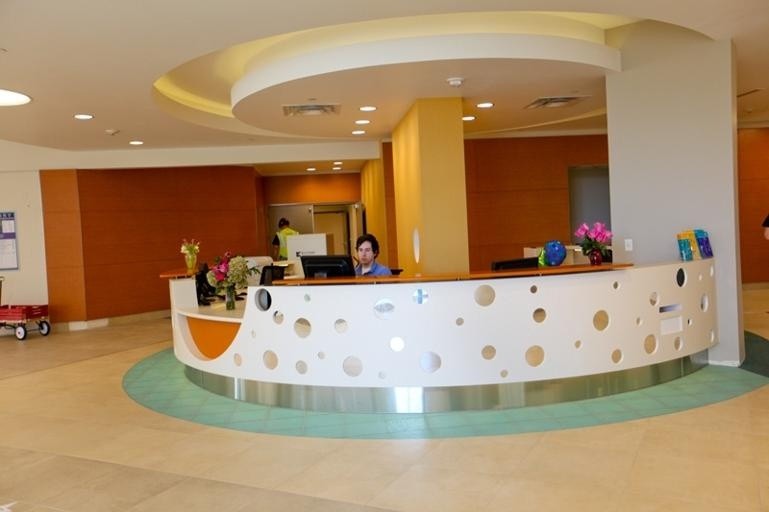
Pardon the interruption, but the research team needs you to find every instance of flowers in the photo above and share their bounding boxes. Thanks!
[574,222,616,257]
[205,250,261,310]
[179,239,200,268]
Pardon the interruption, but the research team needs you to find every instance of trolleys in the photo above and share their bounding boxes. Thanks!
[1,277,52,340]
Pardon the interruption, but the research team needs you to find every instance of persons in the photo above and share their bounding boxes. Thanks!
[352,233,392,277]
[271,217,300,262]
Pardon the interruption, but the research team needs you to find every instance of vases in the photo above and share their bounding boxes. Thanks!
[225,290,236,310]
[185,255,197,270]
[588,247,603,266]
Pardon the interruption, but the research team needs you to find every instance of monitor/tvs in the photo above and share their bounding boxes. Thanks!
[202,261,217,294]
[300,255,356,278]
[492,258,538,270]
